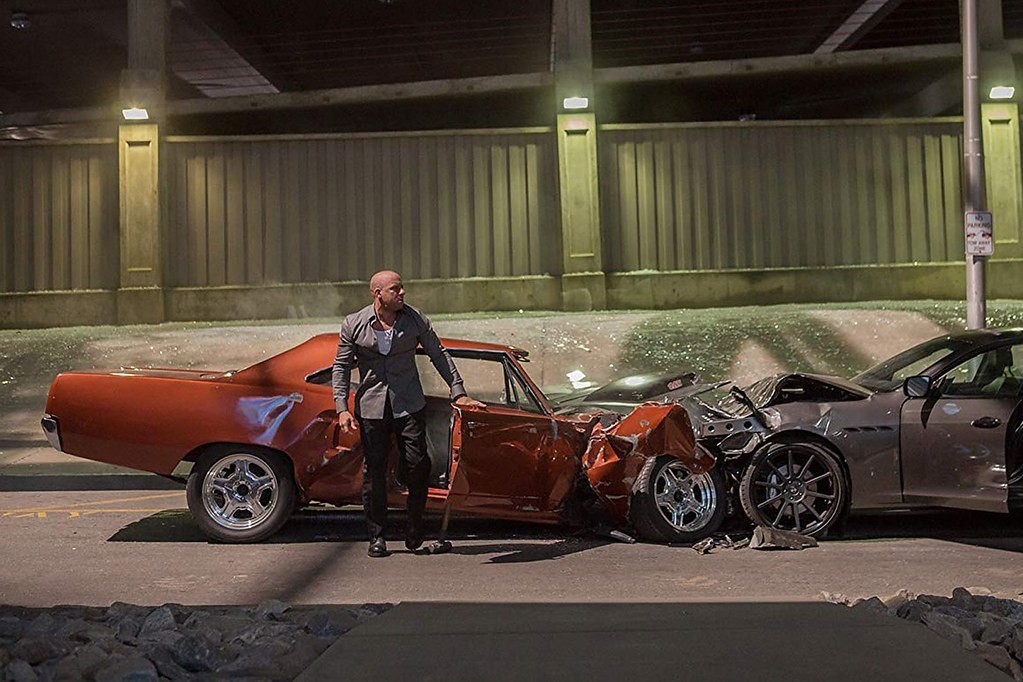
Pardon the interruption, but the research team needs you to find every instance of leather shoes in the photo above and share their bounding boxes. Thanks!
[404,511,425,549]
[368,536,387,557]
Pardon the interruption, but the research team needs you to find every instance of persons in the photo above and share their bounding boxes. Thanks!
[333,269,488,558]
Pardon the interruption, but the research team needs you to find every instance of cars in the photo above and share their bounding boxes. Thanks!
[40,328,1023,546]
